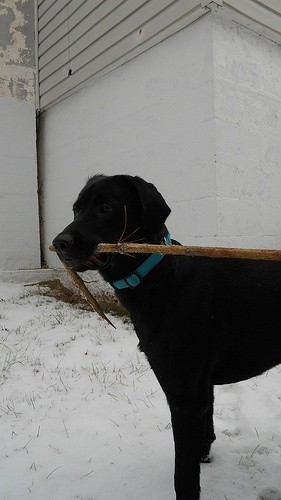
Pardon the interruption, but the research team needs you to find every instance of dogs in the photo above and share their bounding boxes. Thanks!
[53,172,281,500]
